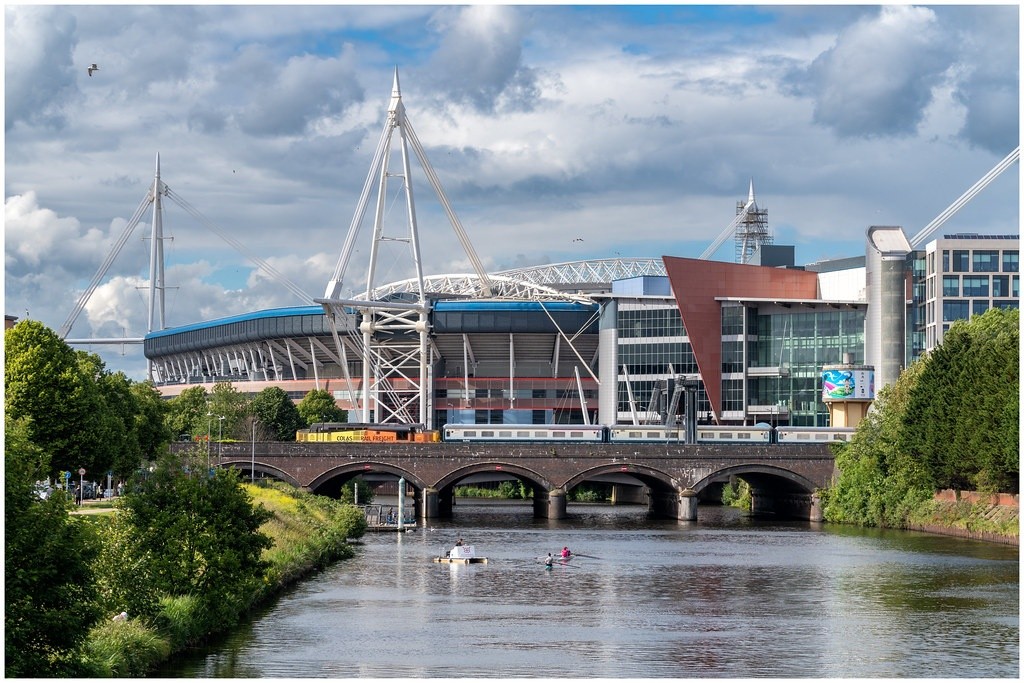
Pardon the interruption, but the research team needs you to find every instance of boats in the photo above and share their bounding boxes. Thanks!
[362,504,417,531]
[559,555,575,561]
[432,544,488,568]
[545,565,552,570]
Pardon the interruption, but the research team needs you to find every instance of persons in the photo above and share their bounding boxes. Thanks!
[562,547,571,557]
[387,507,393,515]
[97,484,105,501]
[545,553,553,566]
[74,488,81,506]
[456,539,462,546]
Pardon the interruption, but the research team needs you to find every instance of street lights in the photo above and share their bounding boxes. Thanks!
[206,412,214,472]
[217,415,226,466]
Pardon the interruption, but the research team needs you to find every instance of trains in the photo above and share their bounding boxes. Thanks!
[296,421,441,444]
[442,422,857,444]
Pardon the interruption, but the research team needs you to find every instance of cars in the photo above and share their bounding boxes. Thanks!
[29,480,117,504]
